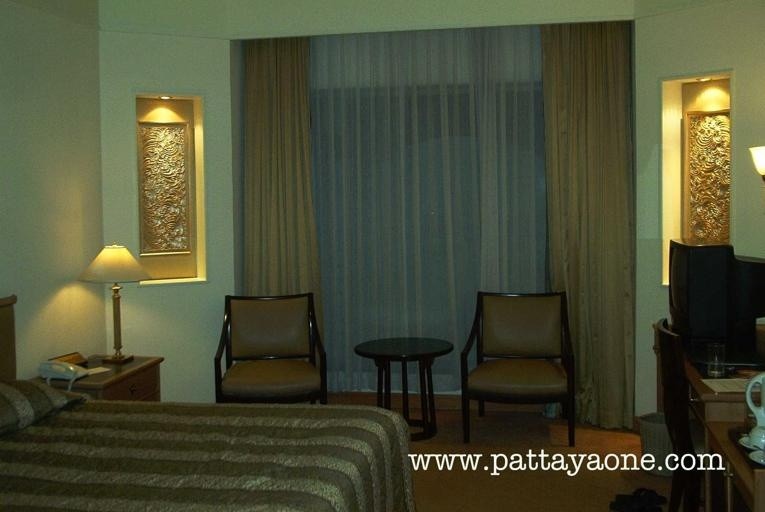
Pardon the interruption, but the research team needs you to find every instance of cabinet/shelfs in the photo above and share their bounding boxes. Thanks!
[703,420,765,511]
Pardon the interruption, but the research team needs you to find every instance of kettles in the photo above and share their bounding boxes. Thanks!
[744,370,765,427]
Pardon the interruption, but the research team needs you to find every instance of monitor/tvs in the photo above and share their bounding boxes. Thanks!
[667,239,765,366]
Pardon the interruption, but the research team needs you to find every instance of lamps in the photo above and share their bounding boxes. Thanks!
[748,144,765,183]
[81,243,154,367]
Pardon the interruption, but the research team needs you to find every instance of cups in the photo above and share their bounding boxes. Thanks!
[749,427,765,448]
[708,343,726,376]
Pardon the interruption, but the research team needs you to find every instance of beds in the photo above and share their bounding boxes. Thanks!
[0,293,420,511]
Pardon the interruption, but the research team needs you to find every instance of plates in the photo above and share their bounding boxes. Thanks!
[749,450,765,465]
[738,437,757,450]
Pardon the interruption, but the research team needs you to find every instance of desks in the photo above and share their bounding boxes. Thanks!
[351,337,454,440]
[650,319,765,511]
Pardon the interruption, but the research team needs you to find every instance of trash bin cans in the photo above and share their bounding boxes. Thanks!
[639,411,675,477]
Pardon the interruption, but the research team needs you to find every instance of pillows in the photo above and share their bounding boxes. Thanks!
[0,372,84,439]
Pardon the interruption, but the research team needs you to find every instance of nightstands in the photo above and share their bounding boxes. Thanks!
[43,349,164,407]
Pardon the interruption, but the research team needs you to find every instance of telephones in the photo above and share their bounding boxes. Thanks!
[38,361,89,380]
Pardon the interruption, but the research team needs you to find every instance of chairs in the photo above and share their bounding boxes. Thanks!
[212,290,335,405]
[652,315,702,512]
[460,290,576,449]
